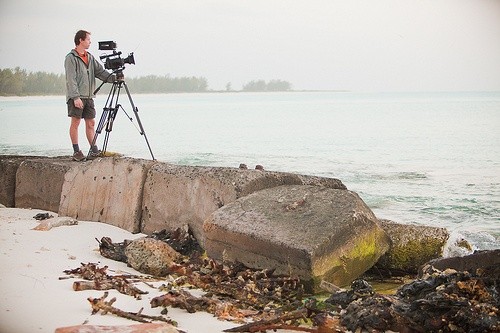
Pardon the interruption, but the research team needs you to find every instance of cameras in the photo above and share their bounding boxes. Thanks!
[98,41,135,71]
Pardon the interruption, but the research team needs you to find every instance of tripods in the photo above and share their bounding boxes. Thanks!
[86,69,155,160]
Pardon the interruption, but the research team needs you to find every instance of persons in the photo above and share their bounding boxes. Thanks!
[62,29,121,161]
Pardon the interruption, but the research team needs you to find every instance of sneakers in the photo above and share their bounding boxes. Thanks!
[86,148,104,162]
[72,150,86,162]
